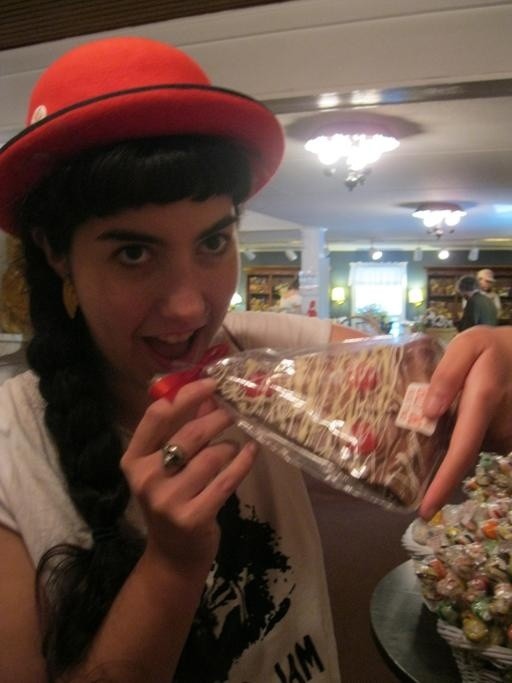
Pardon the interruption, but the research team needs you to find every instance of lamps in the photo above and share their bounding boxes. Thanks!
[236,110,480,264]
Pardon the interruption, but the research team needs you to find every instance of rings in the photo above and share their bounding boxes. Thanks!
[164,441,186,470]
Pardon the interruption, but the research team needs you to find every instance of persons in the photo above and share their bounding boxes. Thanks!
[458,274,498,333]
[463,267,501,311]
[0,38,512,682]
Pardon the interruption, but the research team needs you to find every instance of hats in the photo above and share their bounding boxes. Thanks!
[476,269,497,283]
[0,37,285,239]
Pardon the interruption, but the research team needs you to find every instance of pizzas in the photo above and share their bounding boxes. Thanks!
[202,335,447,506]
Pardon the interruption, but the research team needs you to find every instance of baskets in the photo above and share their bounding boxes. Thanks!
[401,503,512,682]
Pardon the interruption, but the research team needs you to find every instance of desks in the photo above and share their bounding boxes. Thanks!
[365,558,461,683]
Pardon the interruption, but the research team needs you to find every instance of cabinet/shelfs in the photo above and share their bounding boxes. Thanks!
[243,267,303,312]
[425,266,512,345]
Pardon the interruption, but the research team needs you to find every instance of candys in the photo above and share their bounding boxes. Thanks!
[416,452,512,645]
